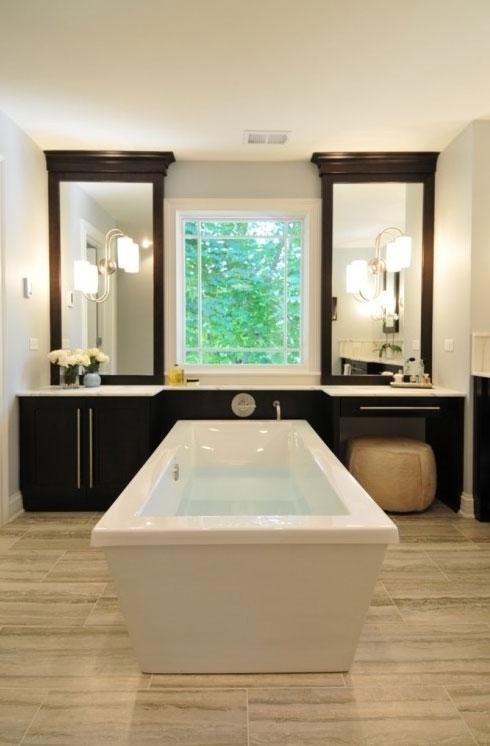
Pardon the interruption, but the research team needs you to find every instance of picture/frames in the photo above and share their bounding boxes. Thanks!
[383,272,398,333]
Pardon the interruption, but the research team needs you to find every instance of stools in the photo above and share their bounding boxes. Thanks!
[346,437,438,512]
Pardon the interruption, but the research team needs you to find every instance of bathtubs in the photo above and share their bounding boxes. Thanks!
[89,418,399,674]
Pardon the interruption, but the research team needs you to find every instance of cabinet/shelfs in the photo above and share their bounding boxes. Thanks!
[19,391,164,512]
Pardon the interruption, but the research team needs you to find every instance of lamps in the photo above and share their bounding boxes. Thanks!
[73,229,140,304]
[346,227,412,316]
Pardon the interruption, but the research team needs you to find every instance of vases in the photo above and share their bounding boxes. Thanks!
[60,367,80,388]
[83,374,101,388]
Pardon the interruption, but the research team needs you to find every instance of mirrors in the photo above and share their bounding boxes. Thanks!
[310,153,439,386]
[44,151,176,385]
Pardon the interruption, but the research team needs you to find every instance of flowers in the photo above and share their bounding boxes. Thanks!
[48,349,81,384]
[82,348,110,373]
